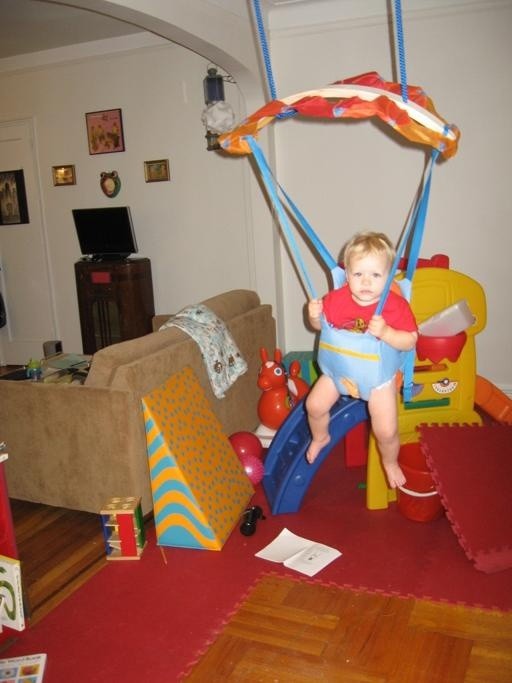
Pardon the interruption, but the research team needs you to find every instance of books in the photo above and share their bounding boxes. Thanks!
[0,653,48,683]
[0,556,26,632]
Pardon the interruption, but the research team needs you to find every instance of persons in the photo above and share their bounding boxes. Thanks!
[304,233,420,488]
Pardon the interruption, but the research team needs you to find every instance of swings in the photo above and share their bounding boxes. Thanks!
[241,134,441,401]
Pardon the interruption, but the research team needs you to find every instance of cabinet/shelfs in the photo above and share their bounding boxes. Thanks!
[75,258,154,354]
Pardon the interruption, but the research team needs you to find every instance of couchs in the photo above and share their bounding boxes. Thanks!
[0,290,277,518]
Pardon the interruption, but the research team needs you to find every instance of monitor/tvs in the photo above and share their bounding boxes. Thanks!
[70,206,139,261]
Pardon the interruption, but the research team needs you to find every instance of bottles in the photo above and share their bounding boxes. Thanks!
[26,359,42,381]
[206,68,225,106]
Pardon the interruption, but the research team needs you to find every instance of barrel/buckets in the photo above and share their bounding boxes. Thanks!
[398,442,443,522]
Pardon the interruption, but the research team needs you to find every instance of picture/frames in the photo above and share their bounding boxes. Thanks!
[143,159,170,182]
[86,109,125,155]
[0,169,29,225]
[52,164,75,187]
